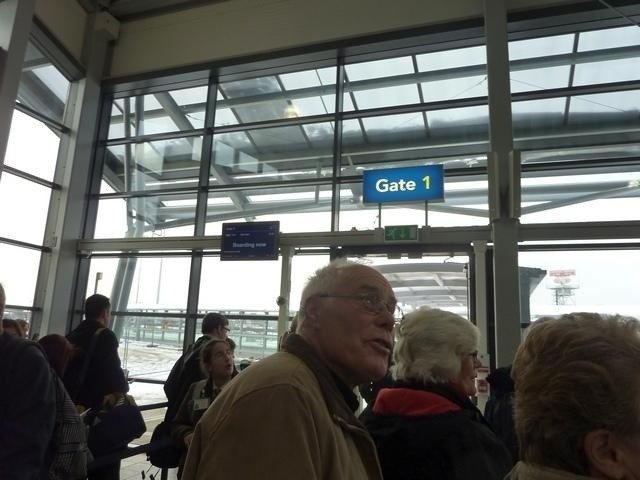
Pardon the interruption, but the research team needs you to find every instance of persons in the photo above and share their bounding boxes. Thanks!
[179,259,404,480]
[163,311,239,480]
[506,312,640,480]
[357,305,520,480]
[0,283,130,480]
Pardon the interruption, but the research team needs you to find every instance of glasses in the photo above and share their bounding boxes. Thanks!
[319,291,400,320]
[468,349,478,361]
[222,326,230,334]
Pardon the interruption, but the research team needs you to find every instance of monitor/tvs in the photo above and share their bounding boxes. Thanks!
[220,221,280,261]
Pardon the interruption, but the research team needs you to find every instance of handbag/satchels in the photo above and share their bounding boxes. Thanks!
[0,335,93,480]
[87,402,147,459]
[149,420,181,468]
